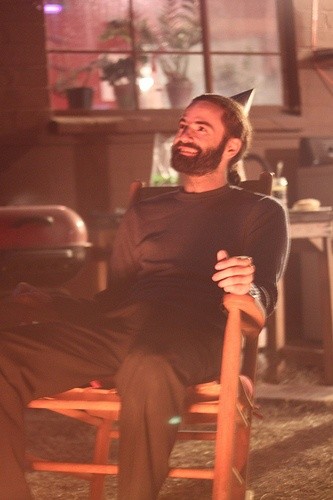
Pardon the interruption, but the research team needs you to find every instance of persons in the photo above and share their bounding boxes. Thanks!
[1,94,289,500]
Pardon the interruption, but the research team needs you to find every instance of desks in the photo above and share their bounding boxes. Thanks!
[265,206,333,385]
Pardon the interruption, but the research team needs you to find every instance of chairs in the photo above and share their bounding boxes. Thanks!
[21,169,275,500]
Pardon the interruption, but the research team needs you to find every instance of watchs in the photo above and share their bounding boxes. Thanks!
[249,282,260,301]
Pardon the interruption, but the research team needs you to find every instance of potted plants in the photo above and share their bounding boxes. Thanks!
[97,8,159,113]
[37,51,115,112]
[153,1,202,109]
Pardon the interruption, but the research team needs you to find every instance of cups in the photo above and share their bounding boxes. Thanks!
[272,176,287,206]
